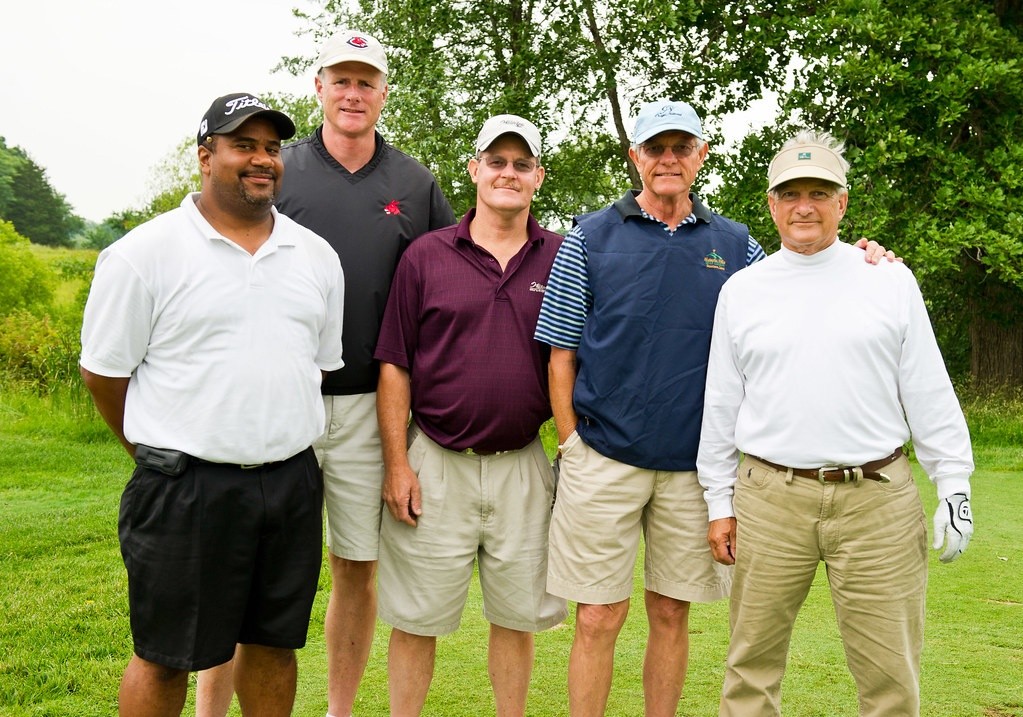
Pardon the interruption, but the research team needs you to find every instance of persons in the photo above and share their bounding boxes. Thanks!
[533,101,903,717]
[695,132,975,717]
[195,31,456,717]
[376,115,564,717]
[81,93,346,717]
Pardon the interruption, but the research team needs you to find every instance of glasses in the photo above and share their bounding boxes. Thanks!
[479,156,538,173]
[642,145,702,159]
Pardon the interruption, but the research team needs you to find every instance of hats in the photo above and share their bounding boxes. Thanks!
[476,114,542,163]
[316,31,388,78]
[766,148,847,194]
[632,101,705,144]
[197,93,296,148]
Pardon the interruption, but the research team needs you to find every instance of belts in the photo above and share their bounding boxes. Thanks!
[744,448,904,483]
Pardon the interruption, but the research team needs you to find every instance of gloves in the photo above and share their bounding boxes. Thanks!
[933,494,973,561]
